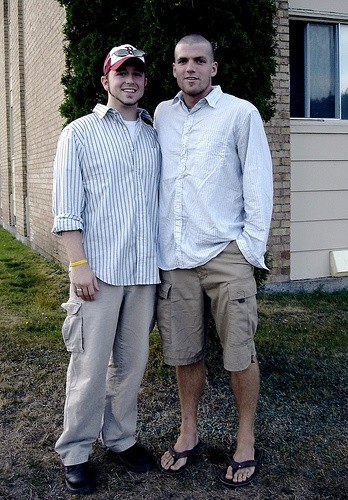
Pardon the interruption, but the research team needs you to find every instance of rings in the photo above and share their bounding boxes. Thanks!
[77,289,82,292]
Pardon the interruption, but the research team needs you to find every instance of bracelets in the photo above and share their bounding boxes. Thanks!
[68,259,88,267]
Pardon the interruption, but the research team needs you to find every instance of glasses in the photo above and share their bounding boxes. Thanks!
[116,48,145,57]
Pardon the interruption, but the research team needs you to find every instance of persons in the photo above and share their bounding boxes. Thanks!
[153,35,274,488]
[51,43,158,494]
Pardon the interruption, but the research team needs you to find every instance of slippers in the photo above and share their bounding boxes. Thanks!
[157,442,204,475]
[218,449,264,487]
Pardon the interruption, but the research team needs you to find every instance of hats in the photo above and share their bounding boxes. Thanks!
[103,45,149,71]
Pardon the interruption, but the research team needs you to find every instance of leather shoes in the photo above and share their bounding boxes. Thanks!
[108,442,157,473]
[64,462,98,494]
